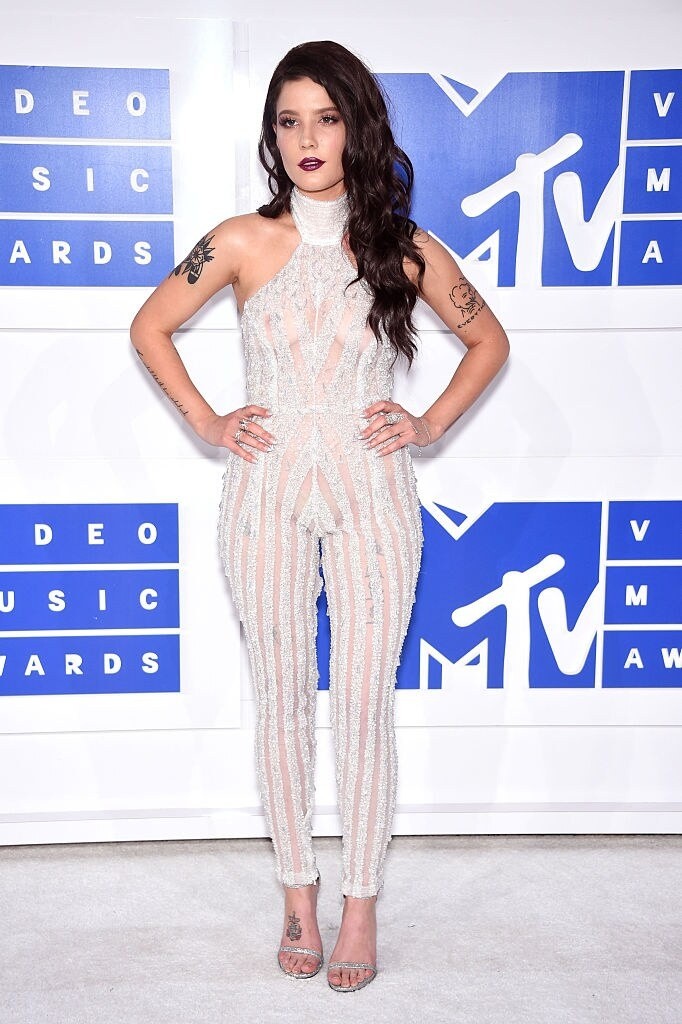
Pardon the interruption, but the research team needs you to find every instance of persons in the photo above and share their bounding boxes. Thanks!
[129,41,511,992]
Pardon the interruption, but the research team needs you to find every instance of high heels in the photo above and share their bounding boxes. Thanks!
[328,962,377,991]
[279,869,324,979]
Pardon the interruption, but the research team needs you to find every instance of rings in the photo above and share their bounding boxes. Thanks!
[239,417,248,430]
[384,410,402,424]
[235,430,243,443]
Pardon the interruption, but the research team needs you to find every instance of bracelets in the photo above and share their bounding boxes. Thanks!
[418,417,430,457]
